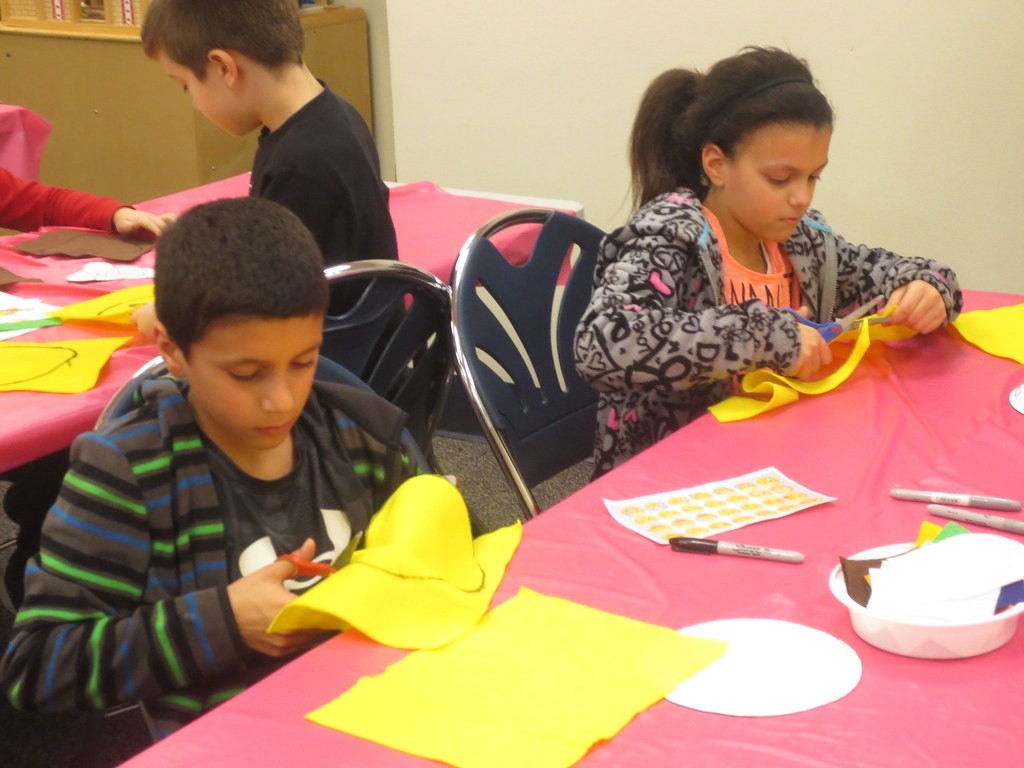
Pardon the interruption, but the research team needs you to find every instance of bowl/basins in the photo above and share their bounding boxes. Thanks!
[829,541,1024,661]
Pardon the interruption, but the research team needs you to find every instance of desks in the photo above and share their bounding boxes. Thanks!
[110,290,1024,768]
[0,170,582,481]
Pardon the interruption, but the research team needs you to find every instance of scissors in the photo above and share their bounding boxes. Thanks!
[275,531,365,580]
[779,295,892,380]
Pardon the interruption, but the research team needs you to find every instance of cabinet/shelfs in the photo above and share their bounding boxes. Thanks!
[0,0,373,208]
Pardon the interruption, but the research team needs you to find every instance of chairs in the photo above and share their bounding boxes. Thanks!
[447,209,608,520]
[318,261,488,541]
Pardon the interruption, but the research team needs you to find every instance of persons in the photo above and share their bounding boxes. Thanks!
[572,45,963,485]
[0,195,435,741]
[0,163,179,618]
[140,1,416,407]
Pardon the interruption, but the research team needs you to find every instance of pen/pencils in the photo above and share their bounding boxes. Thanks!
[668,537,804,565]
[927,504,1024,535]
[891,488,1023,512]
